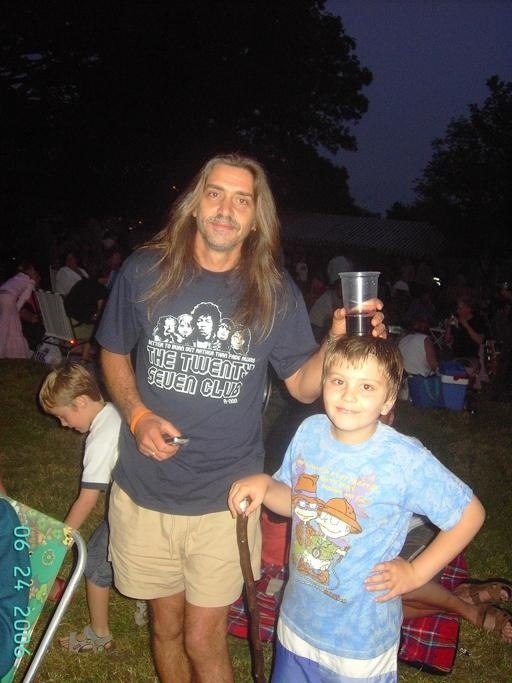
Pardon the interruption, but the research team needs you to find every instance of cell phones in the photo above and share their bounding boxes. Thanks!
[168,436,190,446]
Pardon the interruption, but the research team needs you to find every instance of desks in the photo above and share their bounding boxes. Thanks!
[428,327,453,351]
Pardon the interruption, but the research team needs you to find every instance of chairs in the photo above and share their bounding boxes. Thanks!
[0,491,90,683]
[34,289,93,367]
[49,262,61,291]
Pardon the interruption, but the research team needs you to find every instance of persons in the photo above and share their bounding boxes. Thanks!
[38,364,151,658]
[257,509,512,643]
[1,495,31,678]
[227,334,487,683]
[0,478,77,603]
[94,153,389,683]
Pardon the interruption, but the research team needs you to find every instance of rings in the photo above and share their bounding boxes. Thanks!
[148,452,155,459]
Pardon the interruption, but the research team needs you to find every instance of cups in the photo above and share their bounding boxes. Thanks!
[337,271,381,338]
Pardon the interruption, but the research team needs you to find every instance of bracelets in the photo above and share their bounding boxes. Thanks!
[130,408,151,434]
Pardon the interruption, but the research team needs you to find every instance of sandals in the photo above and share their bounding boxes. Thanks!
[476,602,512,638]
[454,578,512,605]
[58,622,118,654]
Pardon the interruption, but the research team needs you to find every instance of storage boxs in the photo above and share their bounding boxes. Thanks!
[440,370,470,412]
